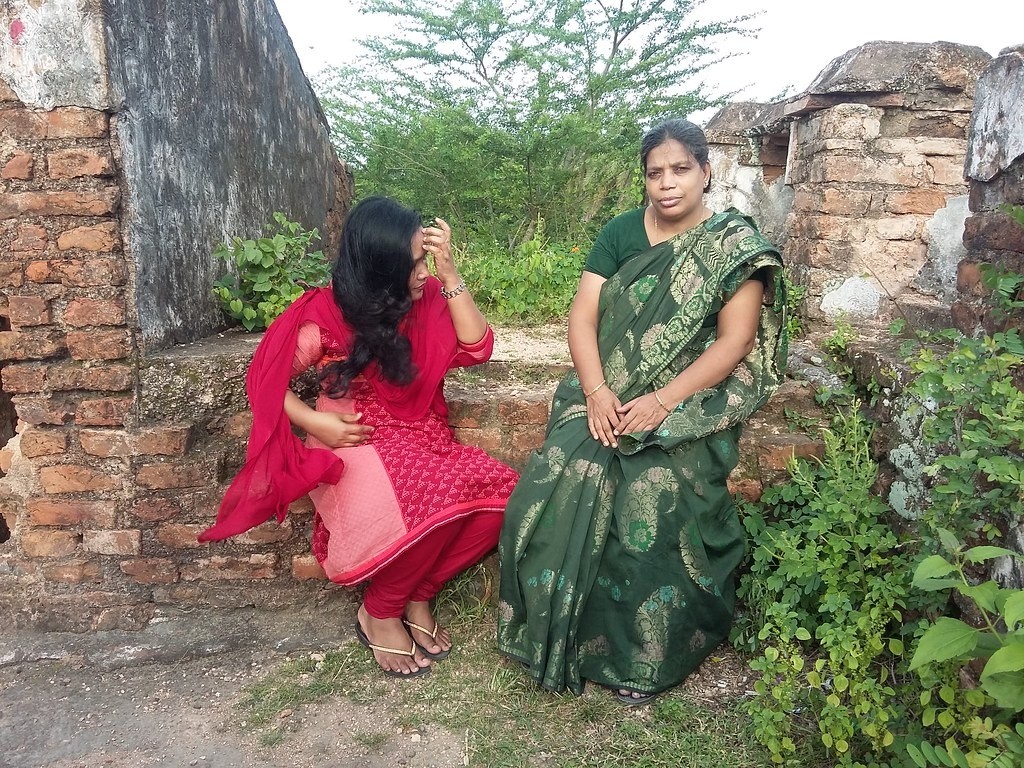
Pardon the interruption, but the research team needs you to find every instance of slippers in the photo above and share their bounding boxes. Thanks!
[355,619,454,679]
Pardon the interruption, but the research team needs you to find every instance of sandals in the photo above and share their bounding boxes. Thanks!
[617,688,657,706]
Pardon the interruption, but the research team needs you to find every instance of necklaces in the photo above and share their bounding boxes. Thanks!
[655,207,705,236]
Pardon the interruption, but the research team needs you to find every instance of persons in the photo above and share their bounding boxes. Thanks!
[497,119,788,705]
[200,195,521,677]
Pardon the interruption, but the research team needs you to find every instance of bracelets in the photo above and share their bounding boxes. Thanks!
[654,390,671,413]
[440,278,465,299]
[585,380,605,398]
